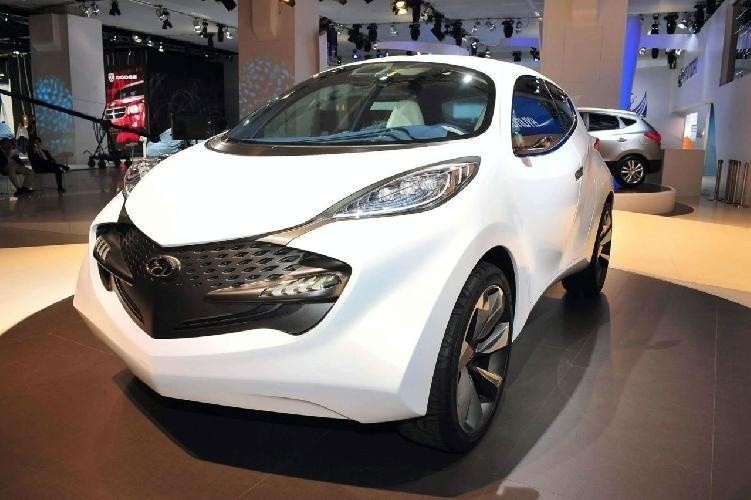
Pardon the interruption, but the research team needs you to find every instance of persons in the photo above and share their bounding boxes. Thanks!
[1,138,34,195]
[28,137,71,193]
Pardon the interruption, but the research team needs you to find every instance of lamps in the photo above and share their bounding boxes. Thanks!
[638,0,719,67]
[392,1,492,59]
[285,1,296,8]
[319,16,378,63]
[110,32,166,53]
[193,17,234,47]
[222,1,238,11]
[512,50,523,62]
[529,47,540,61]
[364,1,372,5]
[109,2,122,18]
[338,1,348,7]
[390,25,400,38]
[77,2,99,19]
[384,50,432,58]
[471,16,525,39]
[154,5,174,30]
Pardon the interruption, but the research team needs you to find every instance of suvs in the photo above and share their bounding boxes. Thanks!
[577,106,669,189]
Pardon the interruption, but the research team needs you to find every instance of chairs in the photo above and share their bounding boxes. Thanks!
[384,99,424,130]
[473,104,521,137]
[0,151,67,197]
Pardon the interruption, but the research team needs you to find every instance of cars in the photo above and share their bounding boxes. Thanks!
[66,51,619,458]
